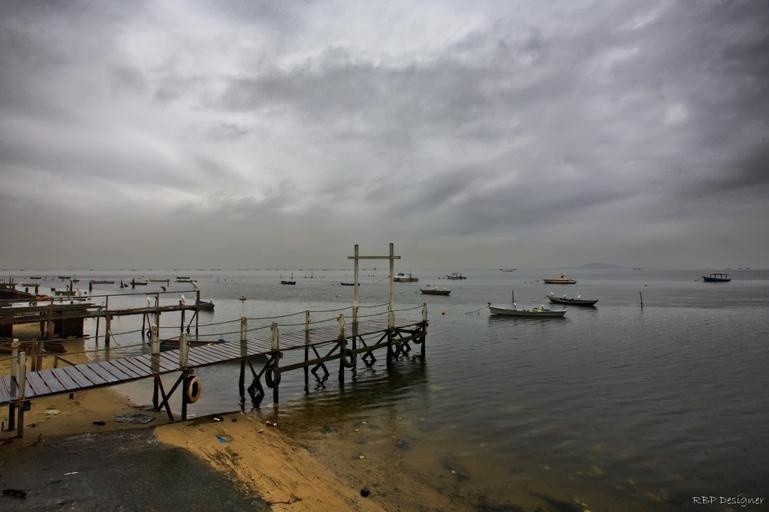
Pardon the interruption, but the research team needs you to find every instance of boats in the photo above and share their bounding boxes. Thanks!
[448,273,467,279]
[340,282,361,286]
[488,290,599,316]
[281,280,297,285]
[393,272,419,282]
[543,273,576,284]
[1,275,198,296]
[703,272,732,282]
[421,287,451,295]
